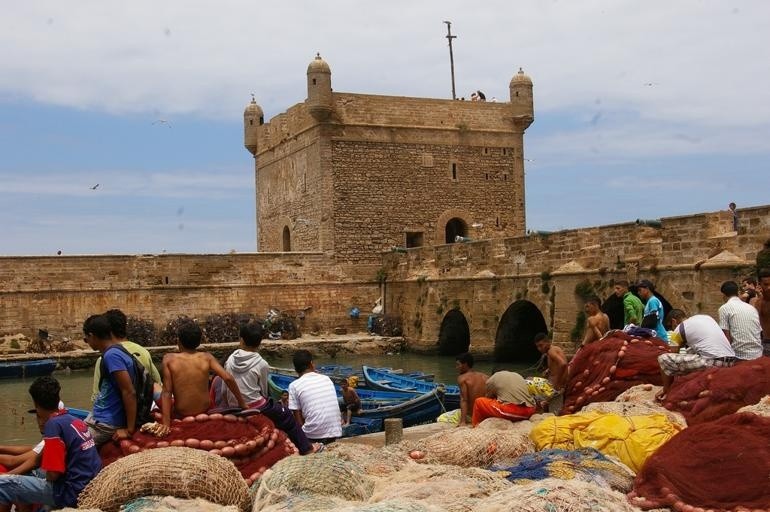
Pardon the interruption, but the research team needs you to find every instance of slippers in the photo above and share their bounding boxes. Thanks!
[305,442,325,455]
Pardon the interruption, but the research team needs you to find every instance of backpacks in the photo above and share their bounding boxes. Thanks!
[98,345,154,431]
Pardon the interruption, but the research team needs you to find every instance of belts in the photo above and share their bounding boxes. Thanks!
[714,358,735,363]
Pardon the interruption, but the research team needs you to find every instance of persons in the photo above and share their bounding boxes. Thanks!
[471,366,536,429]
[728,202,738,231]
[636,278,668,346]
[456,90,498,102]
[151,322,249,435]
[0,309,164,512]
[614,279,645,329]
[208,354,227,411]
[279,390,288,406]
[339,379,362,426]
[524,332,568,408]
[656,308,735,404]
[456,352,488,427]
[718,267,770,366]
[221,322,325,455]
[573,299,610,354]
[288,349,343,445]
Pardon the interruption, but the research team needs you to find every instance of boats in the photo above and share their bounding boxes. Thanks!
[263,361,464,435]
[0,359,58,378]
[26,405,90,424]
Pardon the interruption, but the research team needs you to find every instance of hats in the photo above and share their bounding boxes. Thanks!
[27,399,66,415]
